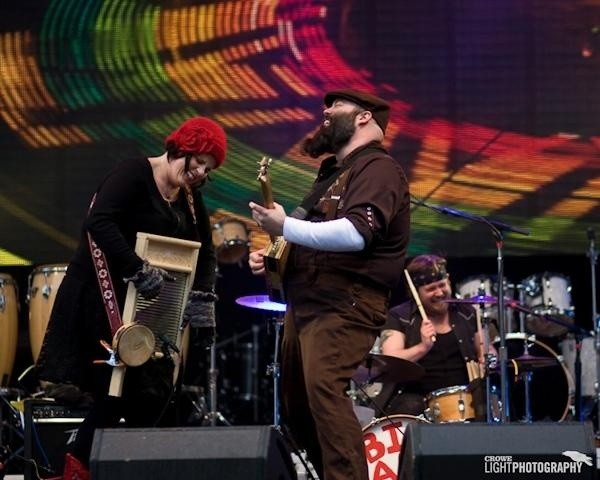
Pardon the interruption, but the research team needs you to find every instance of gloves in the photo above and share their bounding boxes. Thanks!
[180,290,219,348]
[123,258,176,301]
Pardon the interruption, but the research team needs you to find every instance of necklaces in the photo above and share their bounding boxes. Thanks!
[163,189,174,199]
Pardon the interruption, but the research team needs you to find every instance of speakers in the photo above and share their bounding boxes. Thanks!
[24,399,94,480]
[88,425,298,480]
[397,420,599,480]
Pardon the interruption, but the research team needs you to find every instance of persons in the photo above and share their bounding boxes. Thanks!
[376,254,498,418]
[34,117,227,417]
[249,89,410,480]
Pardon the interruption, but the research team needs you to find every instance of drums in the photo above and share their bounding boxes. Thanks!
[29,263,70,365]
[560,333,600,397]
[455,275,518,341]
[211,218,248,263]
[0,273,19,383]
[361,414,431,480]
[522,273,574,338]
[422,384,488,423]
[492,332,574,423]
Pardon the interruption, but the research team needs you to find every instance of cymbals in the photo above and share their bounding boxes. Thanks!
[353,352,422,383]
[505,352,557,371]
[443,294,511,307]
[235,294,286,313]
[525,304,573,337]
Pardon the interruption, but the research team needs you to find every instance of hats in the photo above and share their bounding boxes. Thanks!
[406,255,447,287]
[325,87,390,135]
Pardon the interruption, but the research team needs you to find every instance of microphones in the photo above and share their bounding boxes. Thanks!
[587,225,596,252]
[511,302,543,319]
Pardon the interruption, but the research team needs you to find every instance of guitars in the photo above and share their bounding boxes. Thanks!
[258,156,293,304]
